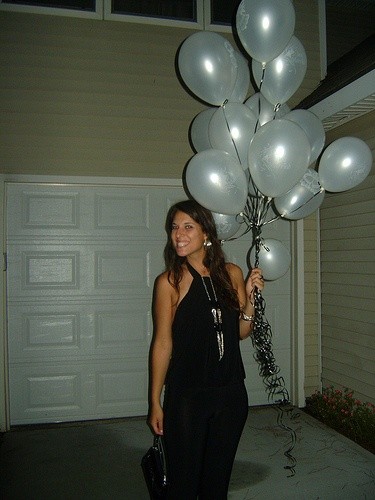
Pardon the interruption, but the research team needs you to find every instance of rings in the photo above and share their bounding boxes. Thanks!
[260,275,264,279]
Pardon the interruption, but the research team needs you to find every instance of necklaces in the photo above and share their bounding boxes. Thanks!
[190,261,224,361]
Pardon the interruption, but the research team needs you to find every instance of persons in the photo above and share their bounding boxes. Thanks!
[148,199,265,500]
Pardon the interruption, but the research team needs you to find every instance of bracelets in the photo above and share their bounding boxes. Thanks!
[242,311,255,321]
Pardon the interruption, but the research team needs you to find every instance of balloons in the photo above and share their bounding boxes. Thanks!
[178,0,372,279]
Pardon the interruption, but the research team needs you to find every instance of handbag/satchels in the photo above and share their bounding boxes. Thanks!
[139,434,178,500]
